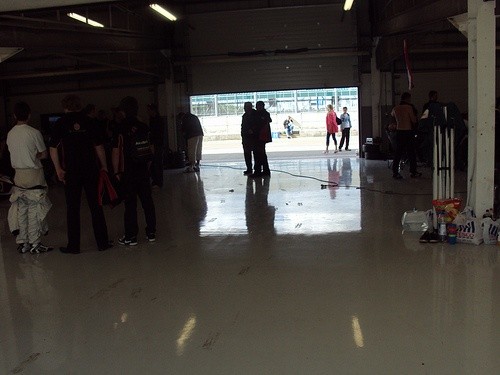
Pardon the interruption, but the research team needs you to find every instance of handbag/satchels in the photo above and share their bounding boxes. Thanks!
[336,116,343,125]
[452,209,500,245]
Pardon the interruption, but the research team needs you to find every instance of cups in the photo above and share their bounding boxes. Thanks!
[438,222,447,242]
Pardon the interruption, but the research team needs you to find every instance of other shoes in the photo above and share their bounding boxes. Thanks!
[145,233,156,243]
[248,172,261,178]
[117,236,138,246]
[261,171,271,175]
[194,167,199,172]
[18,243,31,253]
[244,170,253,174]
[59,246,79,254]
[392,173,403,180]
[419,230,430,242]
[100,240,116,251]
[184,168,194,173]
[429,228,439,242]
[30,245,54,254]
[409,172,422,177]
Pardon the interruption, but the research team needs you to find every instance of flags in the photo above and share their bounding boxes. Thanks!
[404,37,414,90]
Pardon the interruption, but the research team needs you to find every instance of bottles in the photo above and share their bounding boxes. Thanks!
[482,210,492,220]
[490,208,496,222]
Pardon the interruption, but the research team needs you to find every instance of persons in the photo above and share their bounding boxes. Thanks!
[7,194,49,253]
[247,101,272,177]
[241,102,257,174]
[419,90,456,176]
[83,104,96,119]
[177,112,203,173]
[6,102,55,255]
[327,105,337,146]
[391,92,423,179]
[50,94,116,254]
[146,103,166,189]
[493,97,500,219]
[112,95,157,246]
[97,110,112,170]
[339,107,350,151]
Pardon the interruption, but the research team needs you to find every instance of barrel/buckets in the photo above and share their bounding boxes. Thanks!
[174,152,186,169]
